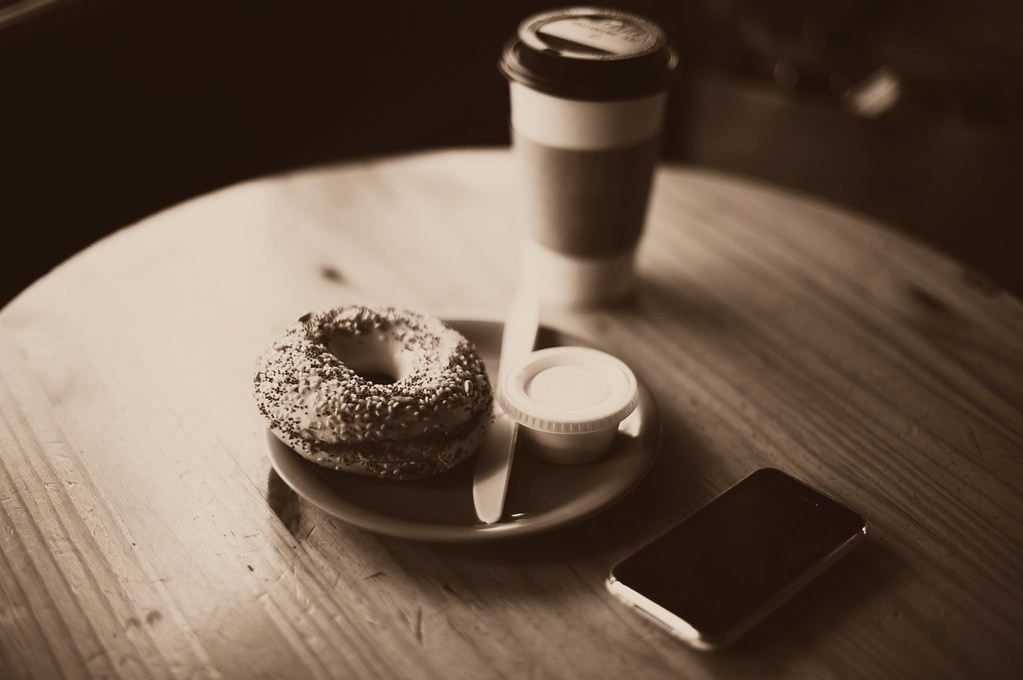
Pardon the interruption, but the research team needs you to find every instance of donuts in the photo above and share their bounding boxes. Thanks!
[255,302,494,484]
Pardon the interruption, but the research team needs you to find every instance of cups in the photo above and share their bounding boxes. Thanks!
[497,6,678,314]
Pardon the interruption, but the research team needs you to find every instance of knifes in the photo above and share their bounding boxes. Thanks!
[472,279,541,525]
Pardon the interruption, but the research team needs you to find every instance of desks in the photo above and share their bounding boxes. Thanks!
[1,146,1022,680]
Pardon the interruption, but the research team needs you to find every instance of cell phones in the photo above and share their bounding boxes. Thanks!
[606,467,869,650]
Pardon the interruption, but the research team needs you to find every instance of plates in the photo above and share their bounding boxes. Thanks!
[262,312,663,543]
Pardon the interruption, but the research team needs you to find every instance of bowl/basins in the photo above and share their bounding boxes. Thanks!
[494,346,640,466]
